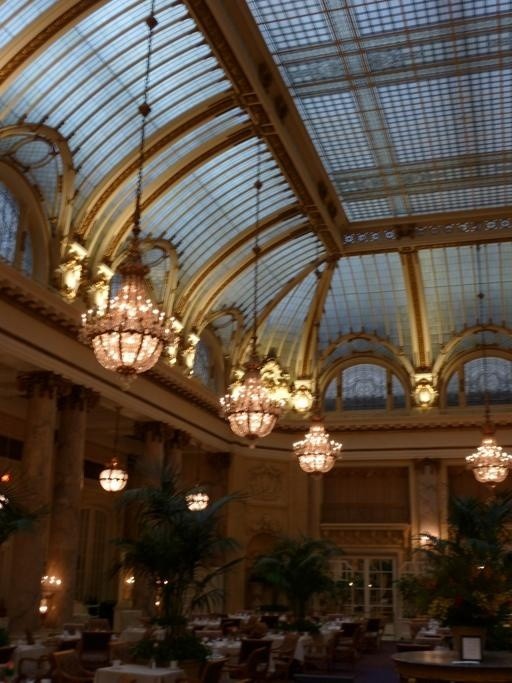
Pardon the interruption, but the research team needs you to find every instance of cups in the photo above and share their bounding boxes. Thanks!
[171,661,177,668]
[113,660,120,669]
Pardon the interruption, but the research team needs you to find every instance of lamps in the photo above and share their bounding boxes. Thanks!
[39,575,63,619]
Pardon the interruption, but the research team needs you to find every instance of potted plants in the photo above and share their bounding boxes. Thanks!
[0,628,17,664]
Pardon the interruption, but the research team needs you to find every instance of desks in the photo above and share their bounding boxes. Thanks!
[391,650,512,683]
